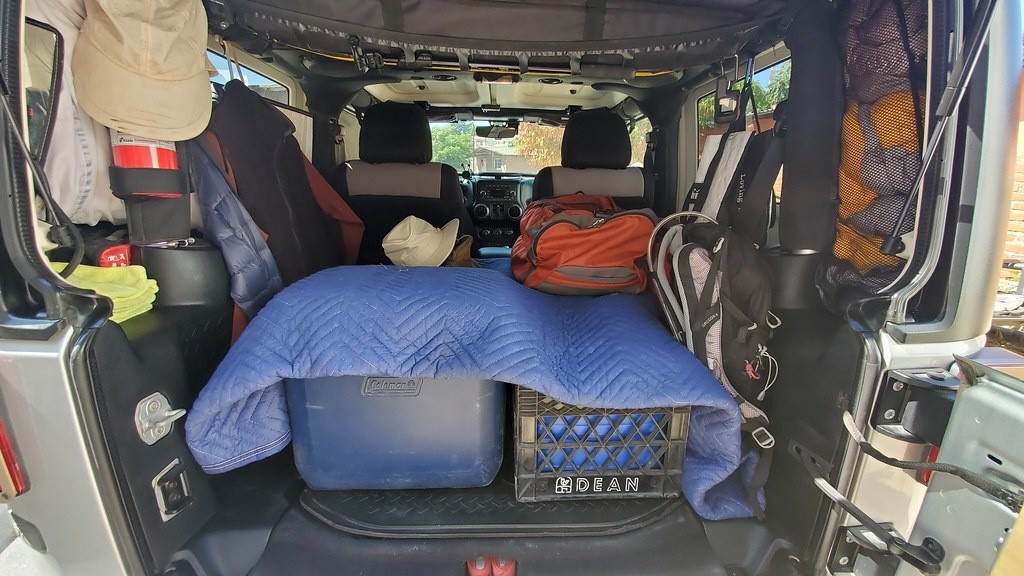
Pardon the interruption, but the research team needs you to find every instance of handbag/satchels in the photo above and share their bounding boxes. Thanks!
[680,85,786,242]
[511,194,659,295]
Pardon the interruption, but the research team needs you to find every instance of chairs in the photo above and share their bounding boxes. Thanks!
[328,103,478,264]
[533,110,655,211]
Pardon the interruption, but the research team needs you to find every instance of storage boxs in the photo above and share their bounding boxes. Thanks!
[512,383,691,502]
[282,378,505,489]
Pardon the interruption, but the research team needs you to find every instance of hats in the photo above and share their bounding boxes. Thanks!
[382,215,459,267]
[71,0,212,141]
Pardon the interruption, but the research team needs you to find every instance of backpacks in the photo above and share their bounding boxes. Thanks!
[645,210,781,451]
